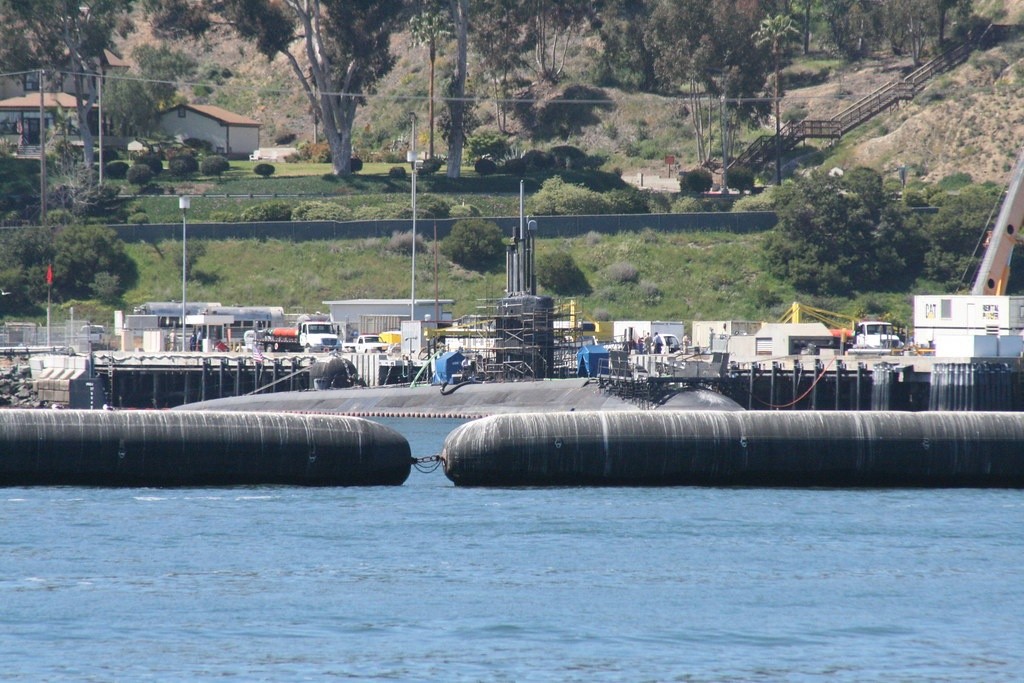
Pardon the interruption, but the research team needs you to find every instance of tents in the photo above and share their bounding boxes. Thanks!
[576,345,610,377]
[433,351,465,384]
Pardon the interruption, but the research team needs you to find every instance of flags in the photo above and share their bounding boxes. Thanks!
[46,265,53,285]
[253,343,265,363]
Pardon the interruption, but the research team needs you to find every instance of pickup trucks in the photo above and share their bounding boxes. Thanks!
[342,335,390,355]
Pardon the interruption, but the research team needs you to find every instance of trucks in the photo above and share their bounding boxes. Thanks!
[846,321,905,350]
[253,315,342,354]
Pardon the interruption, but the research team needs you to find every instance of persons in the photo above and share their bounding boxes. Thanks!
[800,340,817,354]
[624,331,691,354]
[460,357,469,381]
[166,329,203,352]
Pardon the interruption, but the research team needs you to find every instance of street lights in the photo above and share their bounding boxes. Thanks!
[406,108,419,323]
[178,194,192,353]
